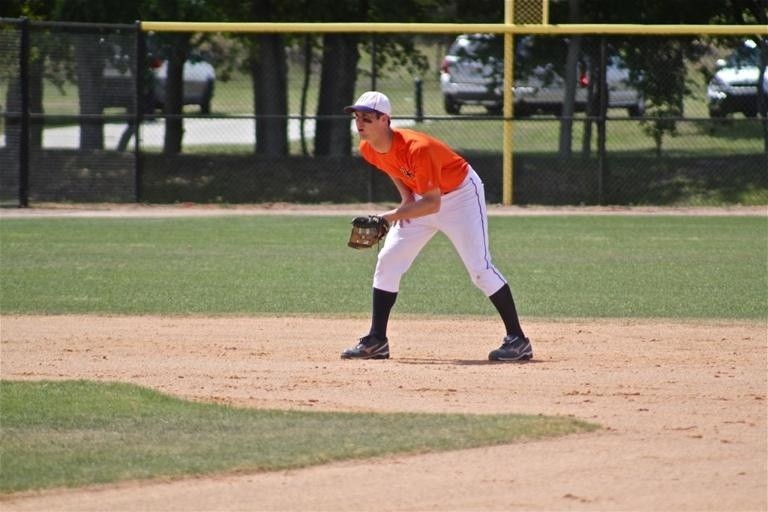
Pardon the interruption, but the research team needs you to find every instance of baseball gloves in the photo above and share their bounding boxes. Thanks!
[348,215,390,249]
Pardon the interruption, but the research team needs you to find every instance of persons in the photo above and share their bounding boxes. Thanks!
[340,90,534,363]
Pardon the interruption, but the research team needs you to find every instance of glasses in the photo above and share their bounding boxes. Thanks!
[352,116,377,123]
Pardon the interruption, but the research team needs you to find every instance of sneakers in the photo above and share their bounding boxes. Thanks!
[489,335,533,361]
[341,334,389,360]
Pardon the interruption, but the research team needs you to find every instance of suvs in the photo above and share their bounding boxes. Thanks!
[94,36,217,119]
[440,31,646,120]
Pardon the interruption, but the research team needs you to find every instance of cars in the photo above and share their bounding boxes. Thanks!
[707,38,768,119]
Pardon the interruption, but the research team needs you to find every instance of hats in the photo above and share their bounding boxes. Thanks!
[344,92,390,115]
